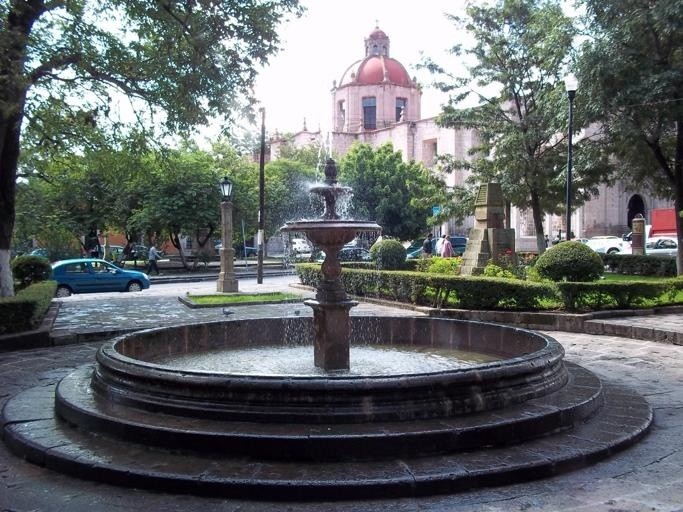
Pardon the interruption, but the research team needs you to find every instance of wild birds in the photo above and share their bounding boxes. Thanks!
[222,307,234,317]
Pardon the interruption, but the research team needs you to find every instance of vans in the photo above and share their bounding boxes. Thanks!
[289,239,312,253]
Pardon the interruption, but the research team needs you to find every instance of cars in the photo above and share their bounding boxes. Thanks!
[580,236,676,259]
[337,248,376,263]
[405,235,466,259]
[214,242,257,258]
[31,243,164,297]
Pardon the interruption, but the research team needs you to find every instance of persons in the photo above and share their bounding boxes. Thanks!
[545,235,549,248]
[422,233,433,258]
[440,235,455,257]
[146,243,164,276]
[435,234,446,257]
[117,239,135,262]
[555,235,559,243]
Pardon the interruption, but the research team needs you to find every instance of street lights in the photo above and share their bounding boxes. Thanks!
[562,73,577,241]
[218,177,235,280]
